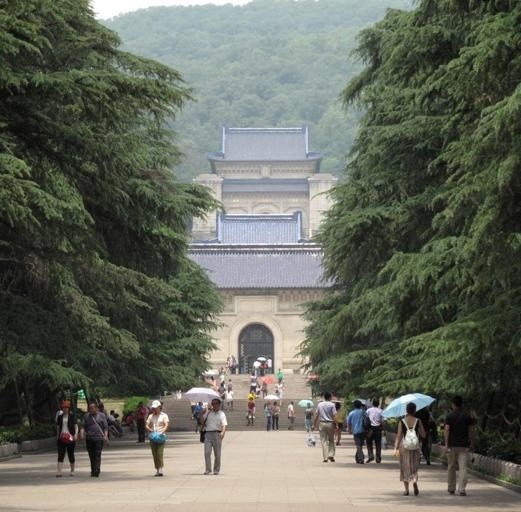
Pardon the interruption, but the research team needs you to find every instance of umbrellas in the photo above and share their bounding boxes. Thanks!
[382,392,436,417]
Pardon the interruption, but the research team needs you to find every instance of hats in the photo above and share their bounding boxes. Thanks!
[149,399,162,409]
[61,399,71,410]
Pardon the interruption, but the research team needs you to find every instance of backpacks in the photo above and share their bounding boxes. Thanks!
[401,417,421,450]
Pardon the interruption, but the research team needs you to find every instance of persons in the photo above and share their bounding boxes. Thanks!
[419,410,436,465]
[79,403,110,478]
[108,401,153,443]
[200,398,228,476]
[393,402,426,495]
[314,392,387,463]
[190,401,210,432]
[207,355,237,413]
[445,395,477,496]
[287,401,295,431]
[145,399,169,477]
[305,403,314,431]
[57,400,80,476]
[55,409,74,424]
[246,356,283,431]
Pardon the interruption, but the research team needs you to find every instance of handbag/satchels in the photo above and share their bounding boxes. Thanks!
[199,430,206,442]
[362,413,371,429]
[148,431,168,444]
[60,432,73,444]
